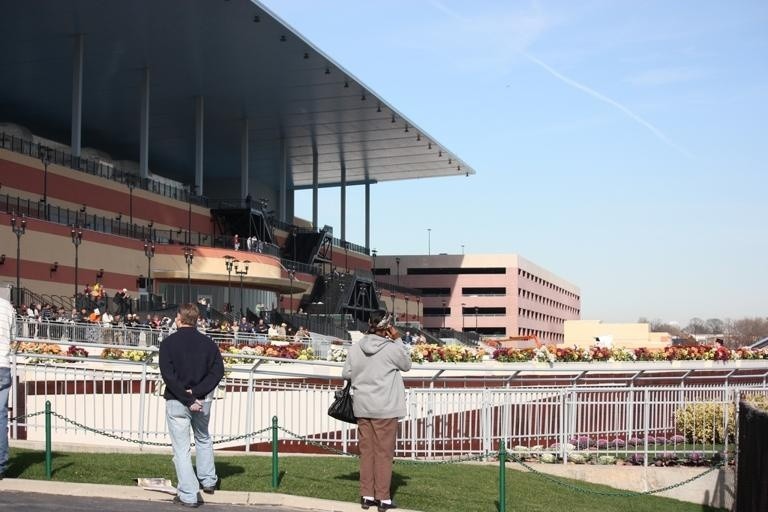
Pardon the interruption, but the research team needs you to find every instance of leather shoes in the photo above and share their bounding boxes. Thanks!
[359,496,380,509]
[378,500,398,512]
[202,484,216,495]
[171,494,199,509]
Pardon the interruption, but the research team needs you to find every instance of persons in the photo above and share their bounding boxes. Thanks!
[81,278,132,313]
[12,298,311,343]
[156,301,223,508]
[404,327,427,343]
[328,310,411,512]
[716,337,725,349]
[245,192,252,208]
[234,231,264,254]
[1,295,14,481]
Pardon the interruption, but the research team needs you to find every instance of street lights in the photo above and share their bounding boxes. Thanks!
[117,171,272,324]
[9,209,26,317]
[283,228,481,340]
[69,222,84,311]
[37,144,55,221]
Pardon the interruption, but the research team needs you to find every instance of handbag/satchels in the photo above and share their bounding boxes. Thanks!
[325,379,362,426]
